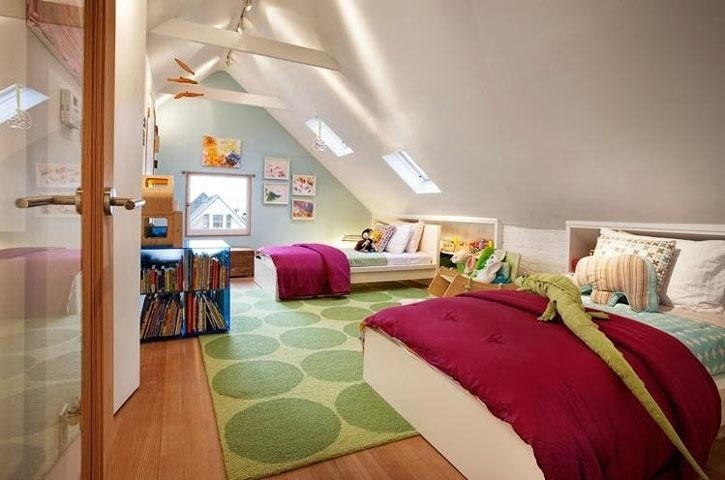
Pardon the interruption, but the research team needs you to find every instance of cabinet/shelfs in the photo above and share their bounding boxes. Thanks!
[137,240,254,346]
[372,216,504,269]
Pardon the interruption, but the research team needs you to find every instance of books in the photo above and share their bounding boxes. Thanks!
[138,246,229,340]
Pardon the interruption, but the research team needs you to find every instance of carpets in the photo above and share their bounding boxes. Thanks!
[198,288,441,479]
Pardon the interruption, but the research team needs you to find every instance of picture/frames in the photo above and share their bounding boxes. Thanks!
[262,155,317,221]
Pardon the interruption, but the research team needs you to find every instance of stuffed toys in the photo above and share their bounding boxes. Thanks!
[359,231,380,253]
[450,245,508,284]
[354,228,377,253]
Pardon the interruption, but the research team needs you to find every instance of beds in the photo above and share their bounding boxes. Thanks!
[140,175,183,248]
[358,220,725,480]
[254,222,441,301]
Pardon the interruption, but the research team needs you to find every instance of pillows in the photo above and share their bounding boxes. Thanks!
[365,219,424,254]
[592,227,725,313]
